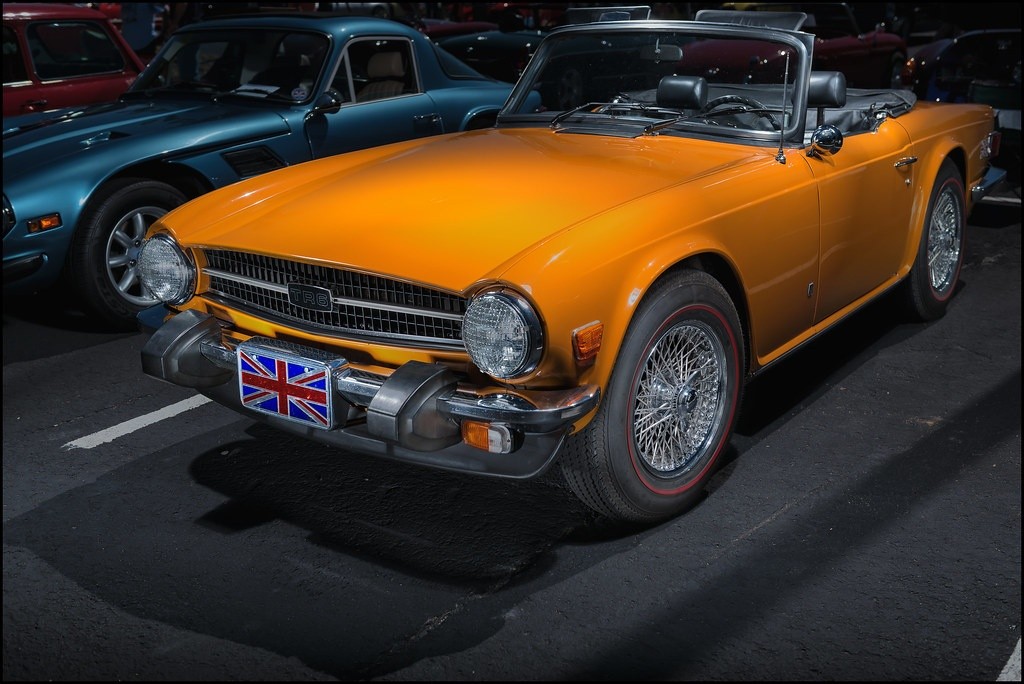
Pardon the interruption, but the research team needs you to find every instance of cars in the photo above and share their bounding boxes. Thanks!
[139,22,1006,523]
[2,0,1024,205]
[3,18,544,325]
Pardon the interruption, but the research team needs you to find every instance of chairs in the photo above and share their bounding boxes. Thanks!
[792,70,846,128]
[356,52,408,103]
[657,76,709,112]
